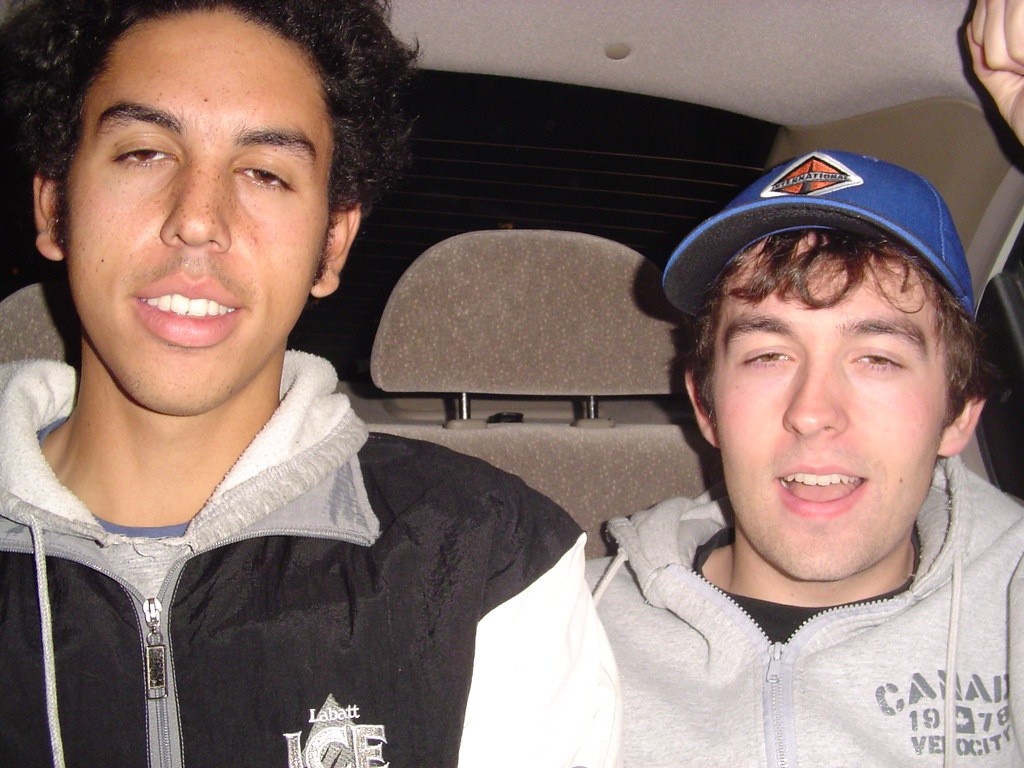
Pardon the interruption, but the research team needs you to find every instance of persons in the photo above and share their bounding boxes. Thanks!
[583,0,1024,768]
[0,0,619,768]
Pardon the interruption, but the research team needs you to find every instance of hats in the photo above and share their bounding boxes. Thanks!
[660,150,974,318]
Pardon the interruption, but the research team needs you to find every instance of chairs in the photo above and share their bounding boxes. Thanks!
[0,279,83,370]
[367,229,723,561]
